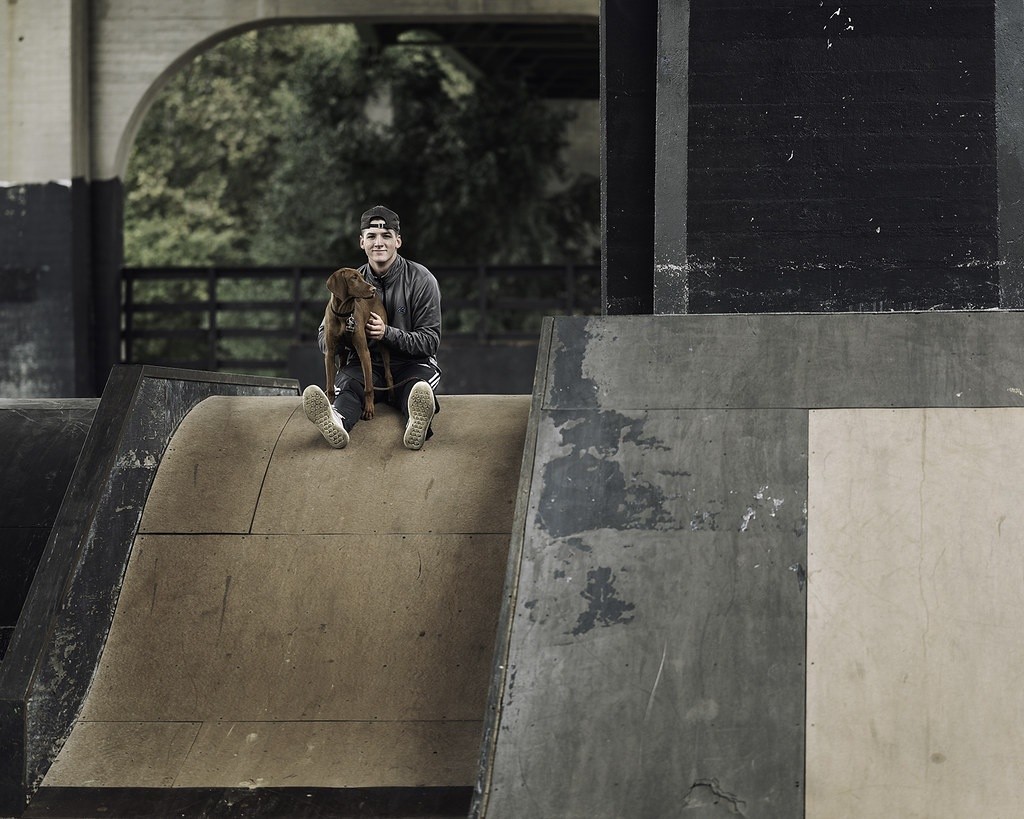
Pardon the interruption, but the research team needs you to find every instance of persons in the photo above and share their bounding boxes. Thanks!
[303,206,441,451]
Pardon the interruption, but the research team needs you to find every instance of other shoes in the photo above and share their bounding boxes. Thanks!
[303,384,349,449]
[403,381,435,450]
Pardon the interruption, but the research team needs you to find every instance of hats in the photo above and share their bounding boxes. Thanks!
[361,206,400,235]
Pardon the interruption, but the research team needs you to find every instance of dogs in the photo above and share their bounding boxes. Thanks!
[324,268,394,421]
